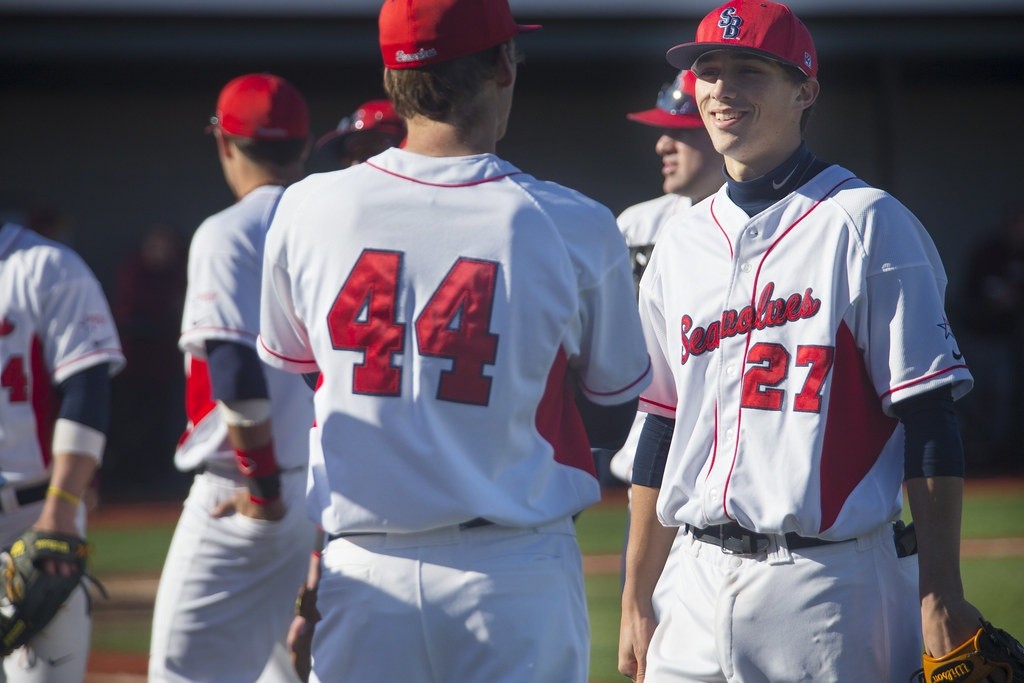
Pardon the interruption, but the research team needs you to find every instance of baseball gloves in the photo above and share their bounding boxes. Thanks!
[920,619,1024,683]
[0,524,93,659]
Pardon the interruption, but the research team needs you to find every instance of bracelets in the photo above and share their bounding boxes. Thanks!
[234,443,277,479]
[249,495,281,504]
[47,487,81,506]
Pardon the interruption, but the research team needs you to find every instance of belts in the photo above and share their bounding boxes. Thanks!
[684,518,857,555]
[0,477,55,513]
[328,517,493,542]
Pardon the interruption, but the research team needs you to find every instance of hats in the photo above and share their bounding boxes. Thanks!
[203,72,310,138]
[627,68,707,128]
[666,0,817,82]
[378,0,542,68]
[314,101,406,155]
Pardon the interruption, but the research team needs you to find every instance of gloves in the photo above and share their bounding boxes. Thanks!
[892,519,918,558]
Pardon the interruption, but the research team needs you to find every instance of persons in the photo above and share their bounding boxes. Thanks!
[0,221,127,681]
[254,1,654,681]
[146,73,406,683]
[616,1,1024,683]
